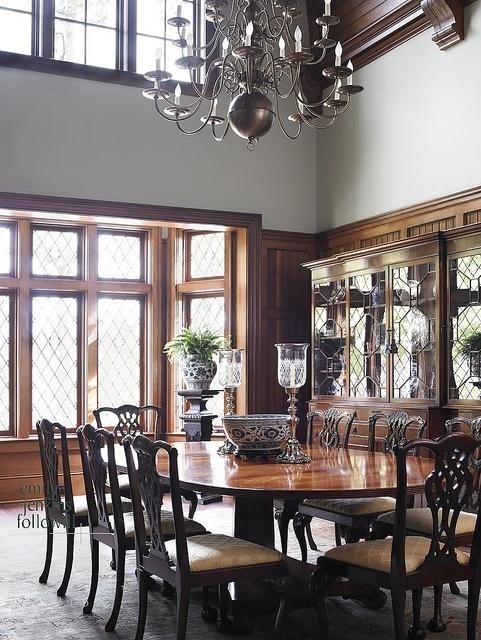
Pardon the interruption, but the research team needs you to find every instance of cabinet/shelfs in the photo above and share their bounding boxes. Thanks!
[300,222,481,509]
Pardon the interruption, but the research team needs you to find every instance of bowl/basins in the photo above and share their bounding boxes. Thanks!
[220,414,301,465]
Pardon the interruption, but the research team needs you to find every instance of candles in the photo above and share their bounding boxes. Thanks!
[290,362,295,386]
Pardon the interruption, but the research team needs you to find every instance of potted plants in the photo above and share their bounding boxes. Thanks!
[456,330,480,375]
[164,329,230,390]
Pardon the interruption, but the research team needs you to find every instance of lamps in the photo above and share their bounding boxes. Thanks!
[142,1,364,150]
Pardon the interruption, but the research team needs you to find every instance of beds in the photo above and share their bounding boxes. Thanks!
[87,441,478,610]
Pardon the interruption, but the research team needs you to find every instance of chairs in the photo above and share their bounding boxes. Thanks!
[122,436,294,639]
[37,420,144,597]
[309,432,481,640]
[76,425,216,632]
[367,417,481,633]
[293,410,427,563]
[93,405,197,519]
[274,408,356,555]
